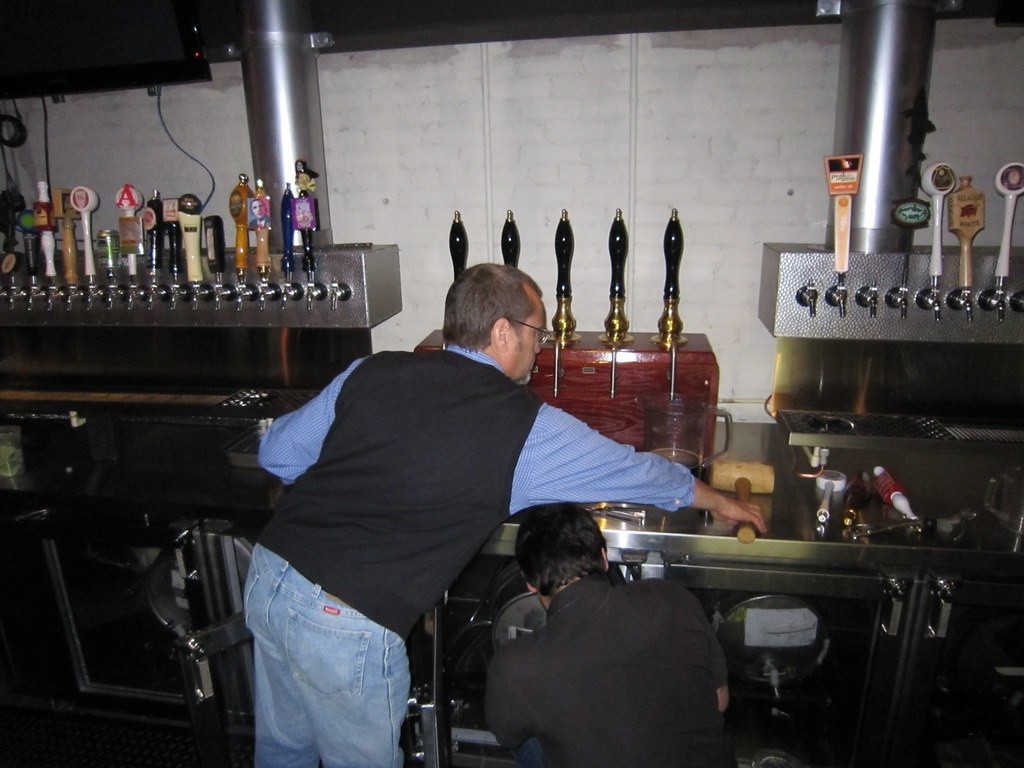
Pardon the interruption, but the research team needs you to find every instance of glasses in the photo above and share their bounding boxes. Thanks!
[491,316,551,345]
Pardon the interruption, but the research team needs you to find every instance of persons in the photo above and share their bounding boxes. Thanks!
[244,263,768,768]
[249,198,271,229]
[484,504,740,768]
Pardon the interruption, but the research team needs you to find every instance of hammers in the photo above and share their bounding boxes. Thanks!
[709,460,775,545]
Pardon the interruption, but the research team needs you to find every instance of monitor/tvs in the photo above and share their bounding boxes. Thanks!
[1,0,213,99]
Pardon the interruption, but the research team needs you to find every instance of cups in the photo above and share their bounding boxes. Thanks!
[0,425,25,478]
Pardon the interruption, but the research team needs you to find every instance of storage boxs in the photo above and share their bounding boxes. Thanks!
[415,328,718,477]
